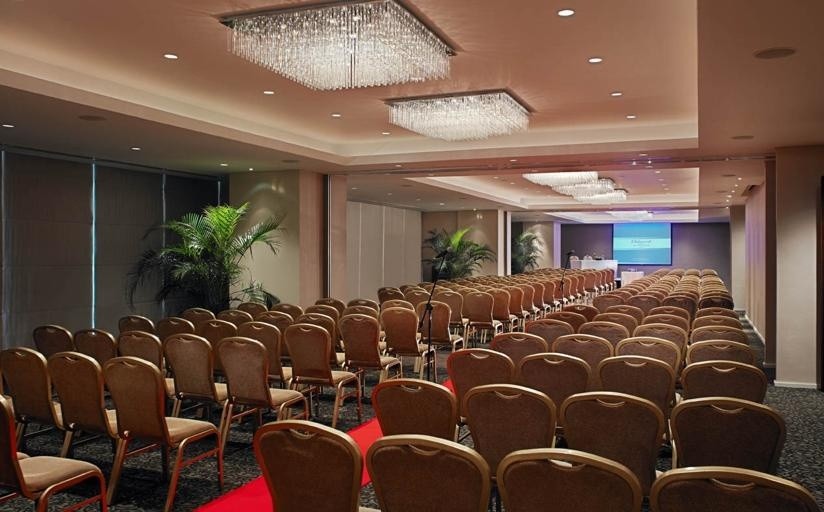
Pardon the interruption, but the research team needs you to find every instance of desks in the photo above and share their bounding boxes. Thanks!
[570,260,617,279]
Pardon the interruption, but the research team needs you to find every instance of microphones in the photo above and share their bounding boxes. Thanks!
[564,249,577,256]
[435,246,454,258]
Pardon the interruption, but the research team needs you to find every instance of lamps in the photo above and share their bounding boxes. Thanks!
[574,188,629,205]
[521,171,599,185]
[216,1,458,91]
[552,178,615,197]
[384,89,530,142]
